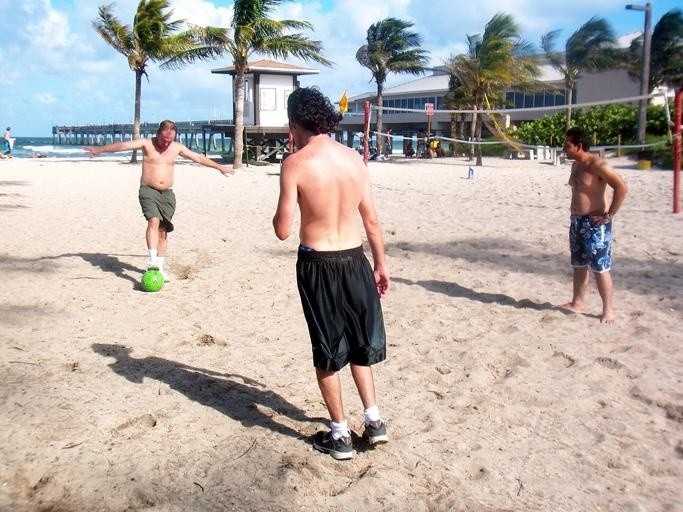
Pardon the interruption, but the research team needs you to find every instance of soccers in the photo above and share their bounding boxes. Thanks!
[141,270,165,292]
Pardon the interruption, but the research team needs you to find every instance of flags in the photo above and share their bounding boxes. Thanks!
[339,91,348,113]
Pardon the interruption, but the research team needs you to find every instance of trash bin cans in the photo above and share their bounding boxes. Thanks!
[638,150,652,170]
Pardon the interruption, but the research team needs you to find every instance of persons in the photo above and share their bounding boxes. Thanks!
[83,120,235,278]
[0,126,12,157]
[271,86,390,459]
[384,126,446,160]
[549,126,628,326]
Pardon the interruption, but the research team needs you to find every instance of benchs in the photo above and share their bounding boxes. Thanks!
[501,144,552,162]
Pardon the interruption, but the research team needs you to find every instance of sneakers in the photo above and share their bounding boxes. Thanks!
[313,431,353,460]
[362,423,389,444]
